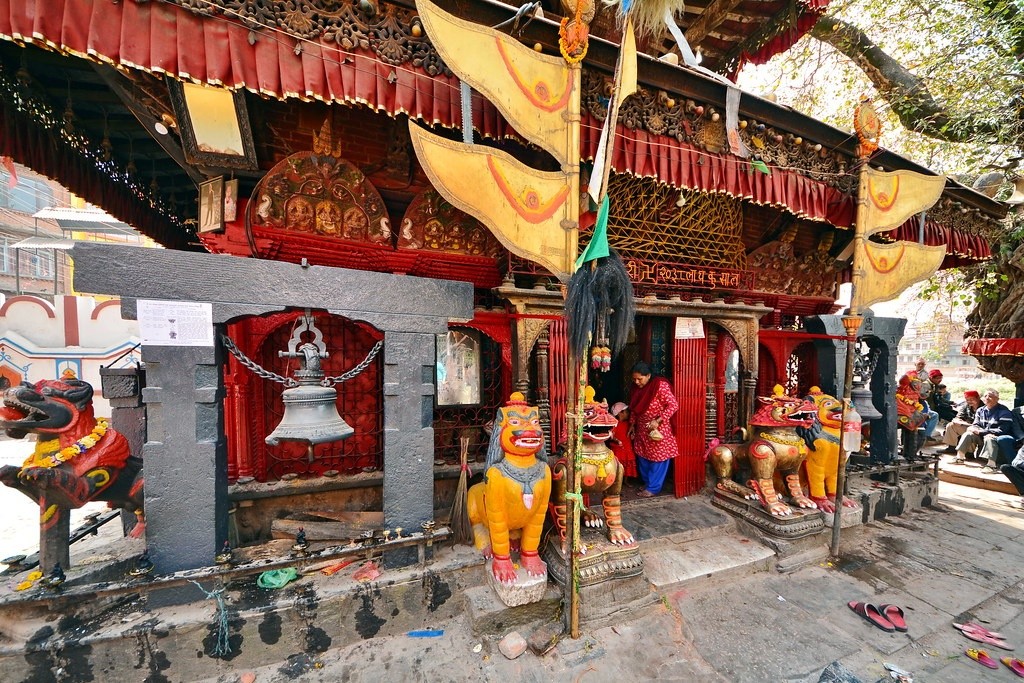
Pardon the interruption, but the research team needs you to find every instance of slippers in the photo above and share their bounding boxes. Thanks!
[879,603,908,631]
[848,601,896,632]
[962,630,1015,650]
[965,648,998,669]
[953,623,1006,641]
[1000,656,1024,678]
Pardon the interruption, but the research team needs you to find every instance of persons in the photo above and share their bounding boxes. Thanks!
[609,402,637,487]
[901,358,1013,474]
[626,361,680,497]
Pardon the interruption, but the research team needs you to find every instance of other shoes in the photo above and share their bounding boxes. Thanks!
[927,435,937,441]
[636,490,655,497]
[639,486,646,491]
[937,448,957,454]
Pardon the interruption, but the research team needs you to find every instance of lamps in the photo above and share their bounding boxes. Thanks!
[155,113,176,135]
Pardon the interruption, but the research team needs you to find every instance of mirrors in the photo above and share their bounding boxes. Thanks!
[437,333,480,405]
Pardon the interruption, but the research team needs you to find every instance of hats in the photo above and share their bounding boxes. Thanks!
[611,402,628,416]
[985,388,999,398]
[929,370,943,378]
[965,391,979,399]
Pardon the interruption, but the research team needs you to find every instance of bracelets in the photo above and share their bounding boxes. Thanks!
[656,419,659,423]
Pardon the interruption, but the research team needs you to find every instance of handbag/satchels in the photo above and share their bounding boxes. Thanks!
[649,428,663,440]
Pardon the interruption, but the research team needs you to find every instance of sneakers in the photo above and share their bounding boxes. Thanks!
[947,457,964,464]
[981,465,997,473]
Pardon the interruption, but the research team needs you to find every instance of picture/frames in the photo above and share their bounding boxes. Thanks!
[165,75,259,171]
[197,175,238,232]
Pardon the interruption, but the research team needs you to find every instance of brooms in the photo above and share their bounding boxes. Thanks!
[436,436,476,553]
